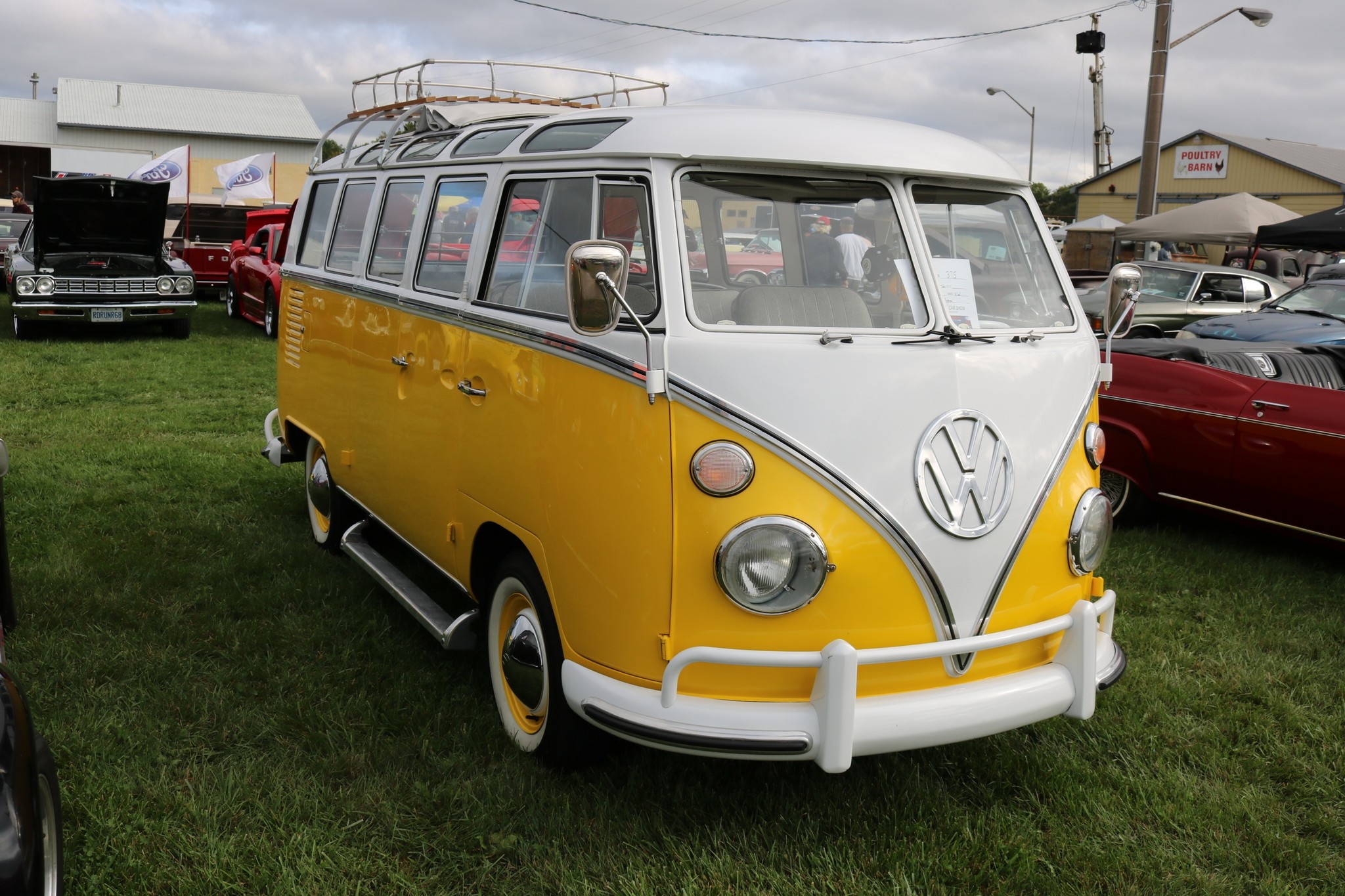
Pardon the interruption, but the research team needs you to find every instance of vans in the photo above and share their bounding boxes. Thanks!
[265,59,1135,776]
[164,197,264,285]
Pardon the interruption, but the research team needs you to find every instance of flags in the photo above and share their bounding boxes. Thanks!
[126,143,188,197]
[211,151,273,208]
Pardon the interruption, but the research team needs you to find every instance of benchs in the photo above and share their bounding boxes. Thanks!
[1211,352,1344,389]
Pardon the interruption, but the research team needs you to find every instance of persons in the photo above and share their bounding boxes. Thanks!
[799,211,872,292]
[1156,239,1174,262]
[10,190,32,238]
[682,208,698,253]
[422,204,531,244]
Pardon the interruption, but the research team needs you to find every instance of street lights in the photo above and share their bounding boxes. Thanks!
[987,87,1035,181]
[1133,8,1274,256]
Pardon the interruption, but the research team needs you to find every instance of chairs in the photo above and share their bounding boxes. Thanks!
[361,260,875,328]
[1198,289,1228,302]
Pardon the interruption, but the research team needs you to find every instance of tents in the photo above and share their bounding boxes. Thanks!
[910,202,1012,237]
[432,194,540,214]
[1047,213,1126,242]
[1107,191,1304,271]
[1247,204,1345,277]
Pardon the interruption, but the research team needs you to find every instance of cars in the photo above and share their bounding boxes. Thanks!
[429,198,1345,546]
[0,176,193,340]
[226,209,293,338]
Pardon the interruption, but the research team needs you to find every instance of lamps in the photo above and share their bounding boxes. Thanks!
[1193,135,1203,144]
